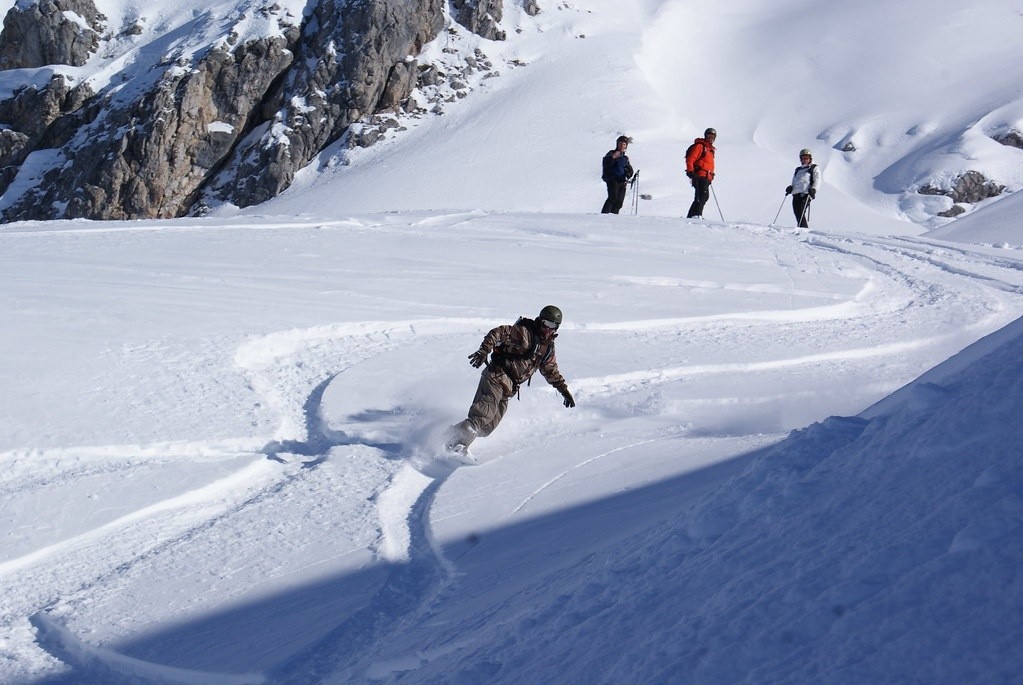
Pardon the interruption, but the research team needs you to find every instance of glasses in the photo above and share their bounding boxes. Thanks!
[801,155,811,159]
[707,134,715,140]
[541,319,557,329]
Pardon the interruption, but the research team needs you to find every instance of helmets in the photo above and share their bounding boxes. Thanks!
[799,149,812,159]
[617,136,628,143]
[539,305,562,324]
[705,128,716,138]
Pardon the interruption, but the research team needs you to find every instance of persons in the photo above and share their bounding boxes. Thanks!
[685,128,716,218]
[601,135,634,214]
[786,149,821,228]
[445,305,576,456]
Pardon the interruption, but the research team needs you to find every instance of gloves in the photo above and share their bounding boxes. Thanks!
[809,190,815,199]
[468,348,489,369]
[561,390,575,407]
[785,186,792,196]
[687,171,695,179]
